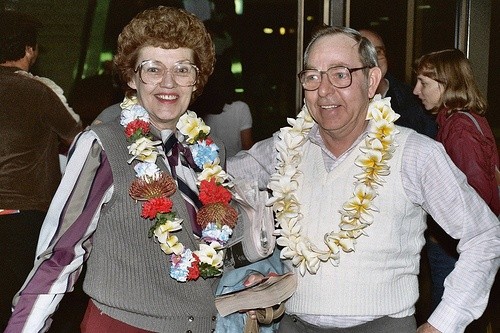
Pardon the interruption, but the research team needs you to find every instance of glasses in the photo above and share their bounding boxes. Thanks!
[134,60,199,86]
[298,66,371,91]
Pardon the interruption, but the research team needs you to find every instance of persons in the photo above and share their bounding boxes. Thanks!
[351,24,439,139]
[413,46,500,333]
[69,74,120,130]
[2,5,238,333]
[226,26,500,333]
[0,8,86,333]
[190,63,253,171]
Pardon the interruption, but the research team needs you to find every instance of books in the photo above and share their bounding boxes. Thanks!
[213,272,298,317]
